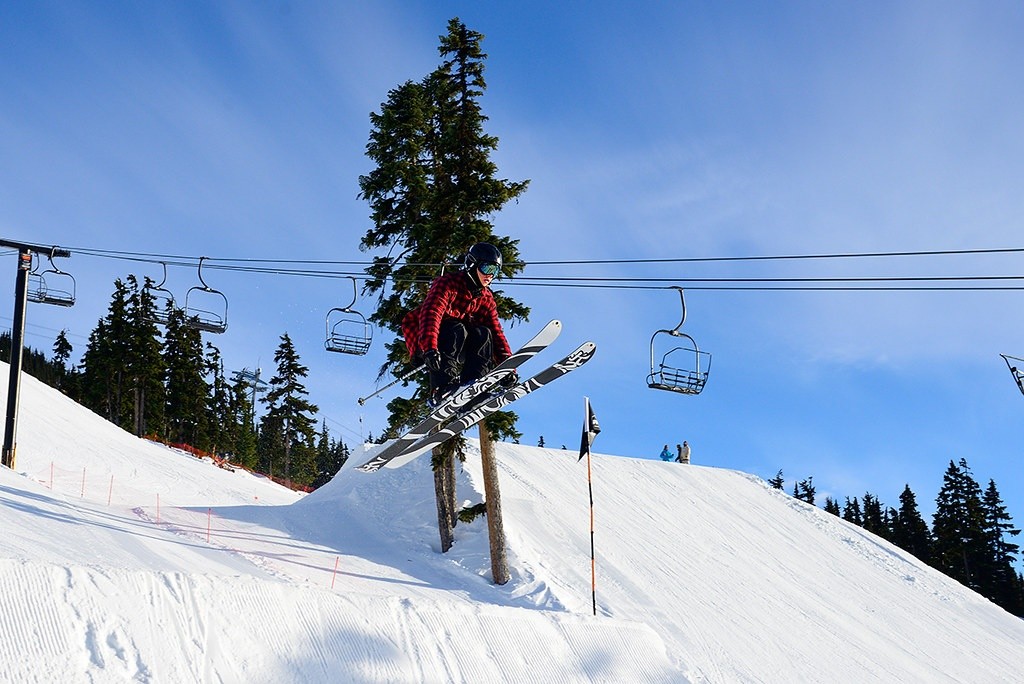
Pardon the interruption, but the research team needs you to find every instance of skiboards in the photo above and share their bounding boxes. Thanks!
[353,318,596,473]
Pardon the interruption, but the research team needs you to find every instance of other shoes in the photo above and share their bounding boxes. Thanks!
[463,379,504,395]
[427,387,453,409]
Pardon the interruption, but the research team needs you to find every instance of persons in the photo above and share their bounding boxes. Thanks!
[660,440,690,465]
[401,243,521,411]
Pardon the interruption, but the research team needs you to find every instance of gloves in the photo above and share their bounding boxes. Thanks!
[422,348,441,372]
[500,373,517,389]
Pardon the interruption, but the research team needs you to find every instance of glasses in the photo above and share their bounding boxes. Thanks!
[477,261,500,279]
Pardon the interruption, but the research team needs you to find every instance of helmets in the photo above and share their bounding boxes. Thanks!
[464,242,502,289]
